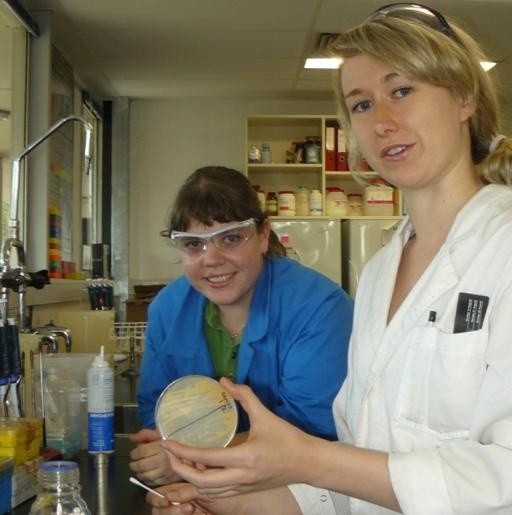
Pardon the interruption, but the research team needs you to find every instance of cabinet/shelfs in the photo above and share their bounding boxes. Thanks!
[243,113,401,220]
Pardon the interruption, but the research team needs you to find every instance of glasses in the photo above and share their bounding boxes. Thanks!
[374,1,458,43]
[170,217,260,258]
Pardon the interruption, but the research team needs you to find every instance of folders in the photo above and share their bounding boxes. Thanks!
[336,126,349,171]
[326,127,338,172]
[359,154,368,172]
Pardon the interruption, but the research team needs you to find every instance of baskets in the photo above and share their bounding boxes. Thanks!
[113,321,149,353]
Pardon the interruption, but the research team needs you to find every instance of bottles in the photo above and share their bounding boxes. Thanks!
[327,178,393,216]
[29,461,92,515]
[88,345,115,455]
[252,185,322,216]
[250,143,271,163]
[286,135,321,164]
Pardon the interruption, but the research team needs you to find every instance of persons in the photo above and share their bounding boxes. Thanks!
[129,165,356,489]
[146,1,511,515]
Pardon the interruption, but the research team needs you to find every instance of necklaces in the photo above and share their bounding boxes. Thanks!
[228,322,246,338]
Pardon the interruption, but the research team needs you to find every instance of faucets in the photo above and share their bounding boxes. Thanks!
[9,114,94,238]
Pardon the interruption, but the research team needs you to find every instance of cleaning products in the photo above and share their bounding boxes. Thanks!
[87,357,117,456]
